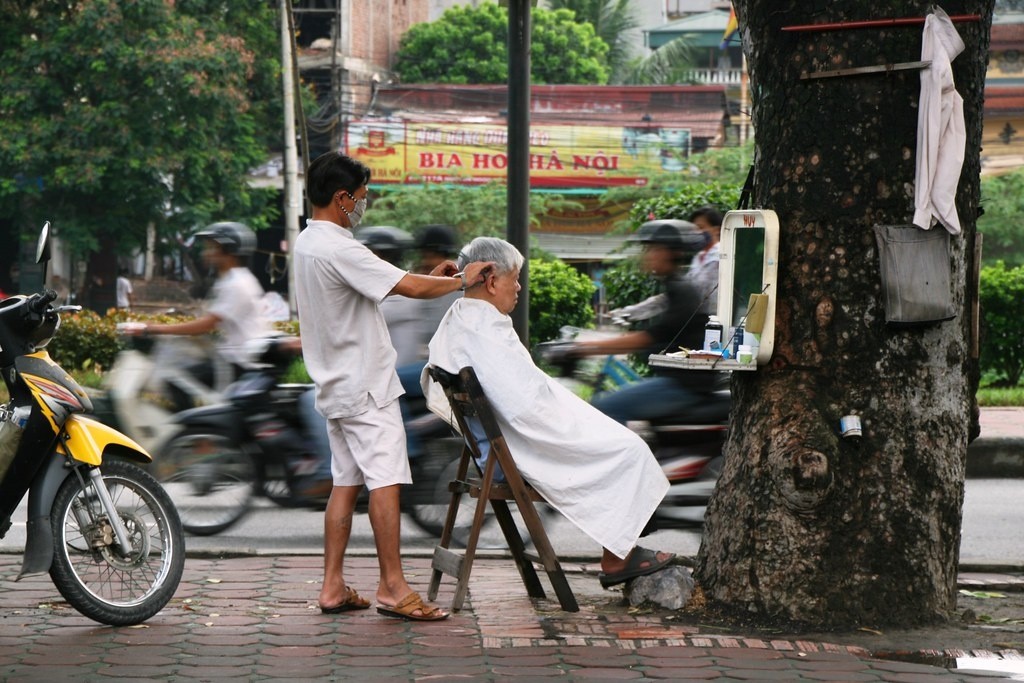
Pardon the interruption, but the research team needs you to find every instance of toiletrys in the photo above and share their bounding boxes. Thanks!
[703,315,723,352]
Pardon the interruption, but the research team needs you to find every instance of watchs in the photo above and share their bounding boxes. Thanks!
[452,271,467,292]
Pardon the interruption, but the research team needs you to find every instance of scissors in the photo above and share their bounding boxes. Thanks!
[456,249,489,283]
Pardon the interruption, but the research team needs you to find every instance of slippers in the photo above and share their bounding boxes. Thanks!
[322,586,371,613]
[599,546,676,587]
[376,592,449,621]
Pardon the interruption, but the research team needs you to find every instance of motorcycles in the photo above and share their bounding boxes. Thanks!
[406,322,733,553]
[76,316,493,537]
[0,221,186,625]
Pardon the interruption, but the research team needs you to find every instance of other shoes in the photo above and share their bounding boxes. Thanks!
[299,479,333,498]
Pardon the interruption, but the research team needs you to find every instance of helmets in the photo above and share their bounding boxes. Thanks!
[626,219,705,264]
[413,224,462,254]
[194,222,257,256]
[355,225,413,248]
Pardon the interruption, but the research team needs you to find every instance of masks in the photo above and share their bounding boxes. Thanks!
[340,192,367,228]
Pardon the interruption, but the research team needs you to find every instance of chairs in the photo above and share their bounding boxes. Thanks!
[428,362,580,614]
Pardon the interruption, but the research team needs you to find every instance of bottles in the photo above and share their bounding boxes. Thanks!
[704,316,723,351]
[733,317,747,360]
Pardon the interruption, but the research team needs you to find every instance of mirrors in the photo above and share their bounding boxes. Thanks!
[716,209,779,368]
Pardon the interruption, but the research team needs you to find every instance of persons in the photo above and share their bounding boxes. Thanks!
[269,222,465,502]
[290,151,493,621]
[550,209,725,429]
[113,268,134,313]
[115,221,274,418]
[424,235,674,586]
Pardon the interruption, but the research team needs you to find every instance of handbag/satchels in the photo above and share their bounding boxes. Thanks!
[873,223,958,322]
